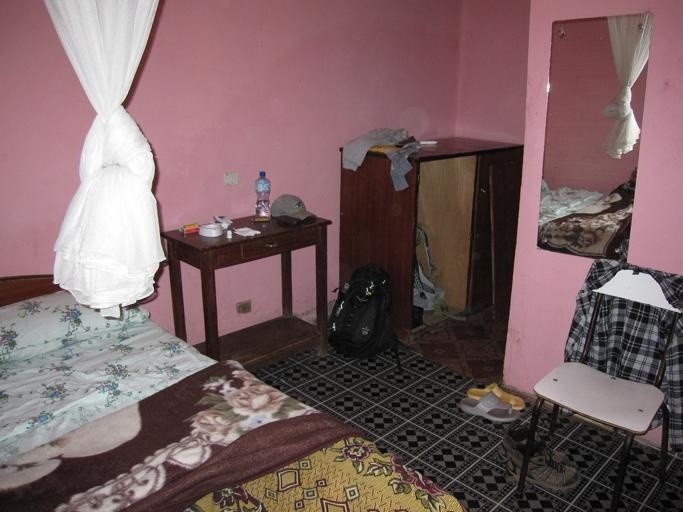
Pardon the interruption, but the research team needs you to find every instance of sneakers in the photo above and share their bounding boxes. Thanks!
[496,428,581,490]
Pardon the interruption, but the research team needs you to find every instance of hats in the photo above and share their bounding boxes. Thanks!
[270,194,315,220]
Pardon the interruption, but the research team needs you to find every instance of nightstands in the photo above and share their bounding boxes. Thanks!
[160,215,332,369]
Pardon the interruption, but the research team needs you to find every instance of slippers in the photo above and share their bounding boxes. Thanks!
[461,393,520,423]
[468,384,524,410]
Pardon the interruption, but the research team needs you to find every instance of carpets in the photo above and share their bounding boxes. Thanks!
[247,342,683,511]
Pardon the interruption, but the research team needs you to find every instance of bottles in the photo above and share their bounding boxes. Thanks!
[254,170,272,218]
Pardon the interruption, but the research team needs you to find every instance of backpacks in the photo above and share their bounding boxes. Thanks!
[330,268,392,357]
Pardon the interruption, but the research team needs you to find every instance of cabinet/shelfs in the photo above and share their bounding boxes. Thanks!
[339,136,524,346]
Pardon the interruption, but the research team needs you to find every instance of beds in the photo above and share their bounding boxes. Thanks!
[0,275,468,512]
[537,166,637,265]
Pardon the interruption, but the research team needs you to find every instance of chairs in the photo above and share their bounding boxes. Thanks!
[517,258,683,512]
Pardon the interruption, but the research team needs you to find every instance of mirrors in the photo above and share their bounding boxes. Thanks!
[536,12,654,264]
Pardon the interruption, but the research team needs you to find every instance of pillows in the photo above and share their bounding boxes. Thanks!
[0,290,150,365]
